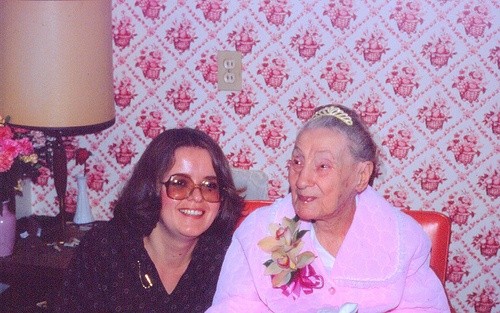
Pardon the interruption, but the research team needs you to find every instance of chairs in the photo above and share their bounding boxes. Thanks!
[233,200,456,313]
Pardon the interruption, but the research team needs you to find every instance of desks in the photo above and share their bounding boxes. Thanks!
[0,214,94,313]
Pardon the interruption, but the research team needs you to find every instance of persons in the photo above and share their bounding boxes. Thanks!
[52,127,249,313]
[204,105,450,312]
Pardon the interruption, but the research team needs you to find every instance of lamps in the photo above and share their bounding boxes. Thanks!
[0,0,116,247]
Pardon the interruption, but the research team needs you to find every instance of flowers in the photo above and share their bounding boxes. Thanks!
[76,148,89,176]
[256,214,324,301]
[0,115,49,202]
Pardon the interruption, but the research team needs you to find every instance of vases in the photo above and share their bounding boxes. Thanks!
[0,200,16,257]
[73,174,95,224]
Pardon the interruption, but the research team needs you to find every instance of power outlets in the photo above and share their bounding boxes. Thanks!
[217,50,242,91]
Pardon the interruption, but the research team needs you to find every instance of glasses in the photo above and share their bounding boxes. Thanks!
[160,173,224,203]
